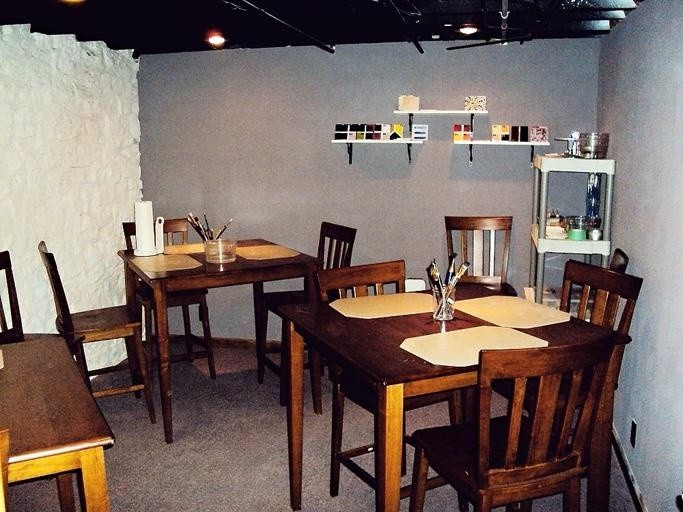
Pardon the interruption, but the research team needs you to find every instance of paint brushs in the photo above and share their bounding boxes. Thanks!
[187,212,233,241]
[426,253,470,320]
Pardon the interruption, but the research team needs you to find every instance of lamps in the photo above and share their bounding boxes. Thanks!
[205,31,226,45]
[460,22,478,35]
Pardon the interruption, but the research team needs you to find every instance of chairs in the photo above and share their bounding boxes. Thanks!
[311,259,462,497]
[444,215,511,288]
[561,259,643,333]
[0,250,93,394]
[265,220,358,414]
[38,240,157,423]
[409,336,609,511]
[122,218,218,380]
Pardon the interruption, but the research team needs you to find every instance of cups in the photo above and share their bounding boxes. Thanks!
[431,287,457,321]
[203,238,237,264]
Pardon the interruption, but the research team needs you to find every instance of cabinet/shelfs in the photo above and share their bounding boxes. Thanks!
[529,155,614,303]
[452,138,550,165]
[391,108,487,136]
[329,138,424,164]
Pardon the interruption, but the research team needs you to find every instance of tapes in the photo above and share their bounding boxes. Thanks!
[567,228,586,241]
